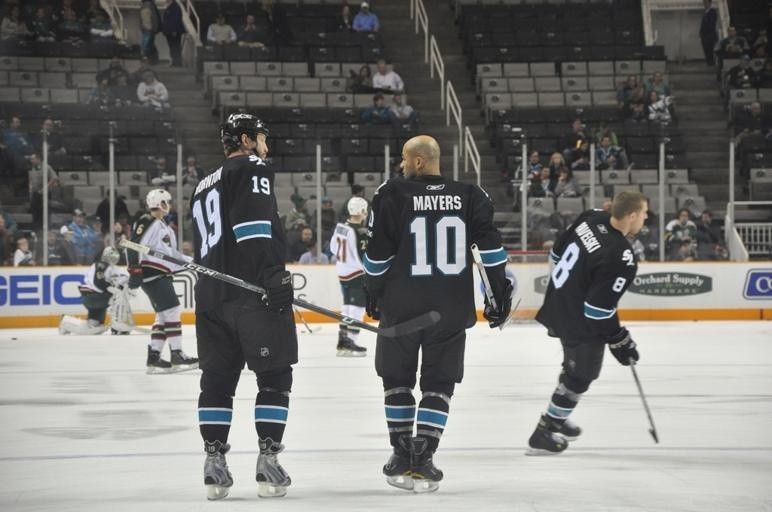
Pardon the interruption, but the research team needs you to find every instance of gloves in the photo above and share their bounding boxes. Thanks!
[608,327,639,366]
[363,286,384,320]
[127,264,142,289]
[483,278,514,328]
[257,271,293,313]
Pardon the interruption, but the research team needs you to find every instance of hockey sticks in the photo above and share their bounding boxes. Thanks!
[293,306,322,337]
[628,355,658,444]
[470,244,521,329]
[120,240,441,338]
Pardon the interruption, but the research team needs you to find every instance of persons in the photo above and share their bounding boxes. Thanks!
[1,116,206,266]
[96,56,170,111]
[327,189,372,354]
[510,111,630,211]
[189,108,298,489]
[501,200,576,251]
[618,69,678,124]
[127,187,201,372]
[523,190,653,456]
[345,58,414,149]
[278,195,337,264]
[363,129,514,481]
[715,26,772,160]
[206,1,308,61]
[699,1,718,66]
[603,201,730,262]
[335,1,379,64]
[59,245,137,337]
[1,1,119,59]
[139,0,184,68]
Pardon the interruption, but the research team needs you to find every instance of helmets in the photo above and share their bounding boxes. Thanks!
[101,246,119,264]
[221,113,270,157]
[348,197,367,215]
[146,189,172,208]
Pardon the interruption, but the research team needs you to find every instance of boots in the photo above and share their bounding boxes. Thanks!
[530,413,581,452]
[171,350,198,365]
[338,332,366,351]
[383,434,412,476]
[410,437,443,481]
[204,440,233,487]
[256,437,291,486]
[147,345,171,368]
